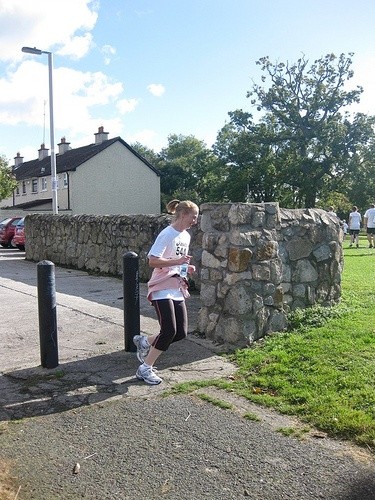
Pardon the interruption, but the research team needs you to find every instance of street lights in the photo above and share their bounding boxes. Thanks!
[21,46,58,216]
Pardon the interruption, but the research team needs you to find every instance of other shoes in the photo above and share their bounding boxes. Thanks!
[369,244,372,248]
[350,245,352,247]
[357,244,358,247]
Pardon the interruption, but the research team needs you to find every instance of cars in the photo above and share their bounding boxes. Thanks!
[13,217,25,253]
[0,216,23,248]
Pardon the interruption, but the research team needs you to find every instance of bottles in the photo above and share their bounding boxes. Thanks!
[180,255,188,279]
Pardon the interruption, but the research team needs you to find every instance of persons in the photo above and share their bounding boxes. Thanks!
[327,206,337,216]
[341,219,348,239]
[133,200,199,385]
[364,203,375,248]
[348,206,362,247]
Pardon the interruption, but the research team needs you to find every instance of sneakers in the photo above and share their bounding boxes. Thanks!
[133,335,152,364]
[135,365,163,385]
